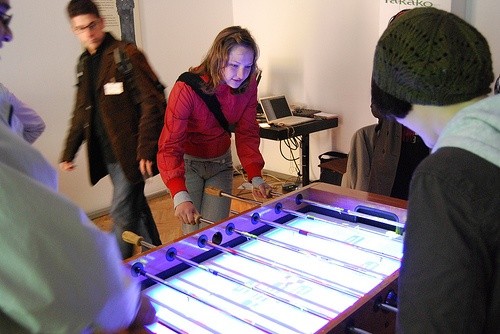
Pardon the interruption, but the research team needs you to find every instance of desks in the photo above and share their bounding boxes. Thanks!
[231,109,338,187]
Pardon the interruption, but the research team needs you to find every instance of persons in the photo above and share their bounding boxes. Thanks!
[370,6,499,334]
[58,0,168,263]
[0,118,158,334]
[0,0,45,145]
[367,96,432,201]
[157,25,275,237]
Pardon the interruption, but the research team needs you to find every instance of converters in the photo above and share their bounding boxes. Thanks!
[282,182,296,190]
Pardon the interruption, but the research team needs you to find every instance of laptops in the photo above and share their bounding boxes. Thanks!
[259,94,314,126]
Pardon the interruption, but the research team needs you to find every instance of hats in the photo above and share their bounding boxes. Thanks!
[371,6,494,104]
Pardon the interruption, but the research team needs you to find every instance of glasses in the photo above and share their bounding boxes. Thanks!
[71,19,101,33]
[0,11,13,26]
